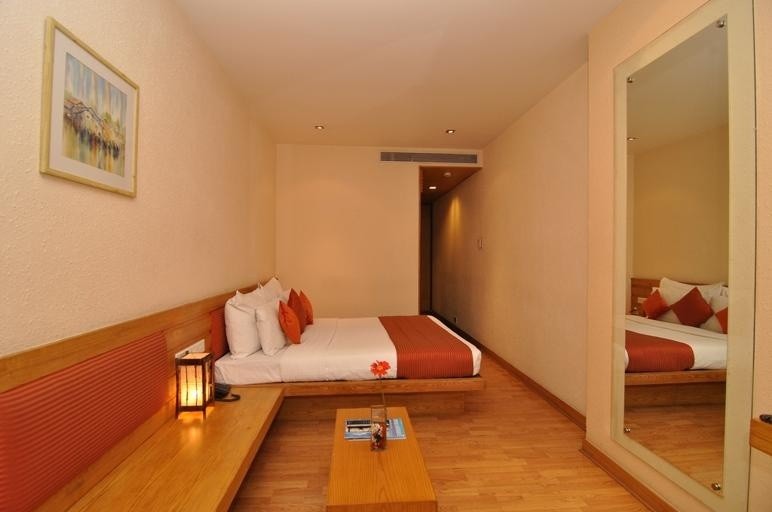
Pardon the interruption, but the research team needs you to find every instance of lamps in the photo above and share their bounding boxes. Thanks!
[174,351,216,421]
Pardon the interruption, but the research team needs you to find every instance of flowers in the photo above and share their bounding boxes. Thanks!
[368,356,392,417]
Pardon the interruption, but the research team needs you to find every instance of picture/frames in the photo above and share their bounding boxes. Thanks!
[38,15,143,199]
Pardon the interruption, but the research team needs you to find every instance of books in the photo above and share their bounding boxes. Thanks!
[344,416,406,441]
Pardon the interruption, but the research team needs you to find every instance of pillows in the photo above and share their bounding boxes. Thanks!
[224,282,268,360]
[640,279,728,336]
[276,296,301,346]
[286,286,306,335]
[298,290,314,325]
[253,305,286,355]
[261,276,284,299]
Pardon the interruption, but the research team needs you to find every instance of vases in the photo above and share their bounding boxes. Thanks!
[368,406,388,450]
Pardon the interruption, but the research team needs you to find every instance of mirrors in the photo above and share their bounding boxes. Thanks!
[610,0,757,510]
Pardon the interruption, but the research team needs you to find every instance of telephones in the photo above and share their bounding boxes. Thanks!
[214,383,231,399]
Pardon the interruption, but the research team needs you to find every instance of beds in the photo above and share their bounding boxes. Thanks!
[624,276,728,414]
[212,277,485,417]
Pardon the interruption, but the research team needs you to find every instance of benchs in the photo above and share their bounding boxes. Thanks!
[1,320,289,510]
